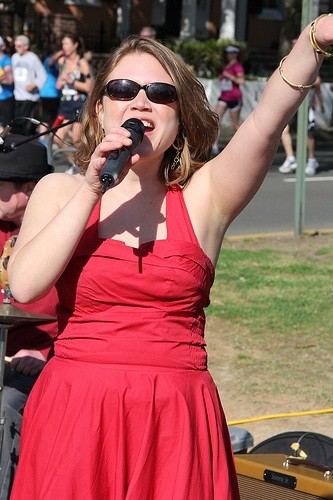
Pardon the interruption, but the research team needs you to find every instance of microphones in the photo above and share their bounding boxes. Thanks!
[99,118,144,193]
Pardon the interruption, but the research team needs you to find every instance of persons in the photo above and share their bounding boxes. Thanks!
[141,27,155,40]
[279,38,324,175]
[0,131,68,455]
[212,46,245,153]
[0,36,96,175]
[8,10,333,500]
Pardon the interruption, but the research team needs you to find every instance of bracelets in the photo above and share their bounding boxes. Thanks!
[279,57,318,89]
[309,14,333,57]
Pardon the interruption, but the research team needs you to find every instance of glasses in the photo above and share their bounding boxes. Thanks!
[101,78,180,105]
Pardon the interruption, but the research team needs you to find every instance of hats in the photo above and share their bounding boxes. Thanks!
[225,45,240,53]
[0,131,55,182]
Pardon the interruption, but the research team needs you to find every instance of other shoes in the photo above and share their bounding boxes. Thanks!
[211,144,219,154]
[67,163,81,174]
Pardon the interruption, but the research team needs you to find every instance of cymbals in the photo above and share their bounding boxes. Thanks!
[1,303,56,325]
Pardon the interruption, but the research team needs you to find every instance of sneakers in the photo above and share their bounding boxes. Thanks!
[305,159,319,176]
[278,157,297,173]
